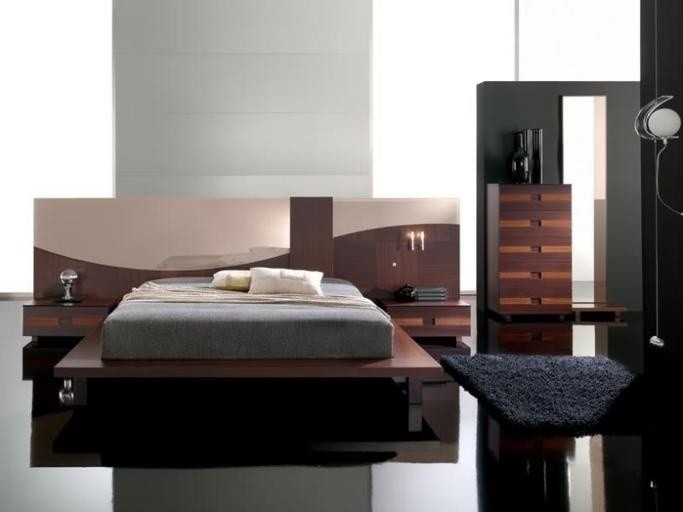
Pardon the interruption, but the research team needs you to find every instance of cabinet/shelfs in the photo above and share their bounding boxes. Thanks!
[484,182,572,321]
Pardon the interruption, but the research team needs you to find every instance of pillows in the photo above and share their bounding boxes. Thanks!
[247,266,324,295]
[210,269,251,290]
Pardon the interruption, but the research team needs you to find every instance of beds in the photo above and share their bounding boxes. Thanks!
[22,196,469,409]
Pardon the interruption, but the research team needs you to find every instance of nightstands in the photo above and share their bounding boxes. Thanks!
[380,297,471,347]
[22,297,118,342]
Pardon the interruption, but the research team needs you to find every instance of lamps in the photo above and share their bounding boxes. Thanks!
[632,95,682,346]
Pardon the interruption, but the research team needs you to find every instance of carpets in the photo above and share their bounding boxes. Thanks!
[440,353,636,425]
